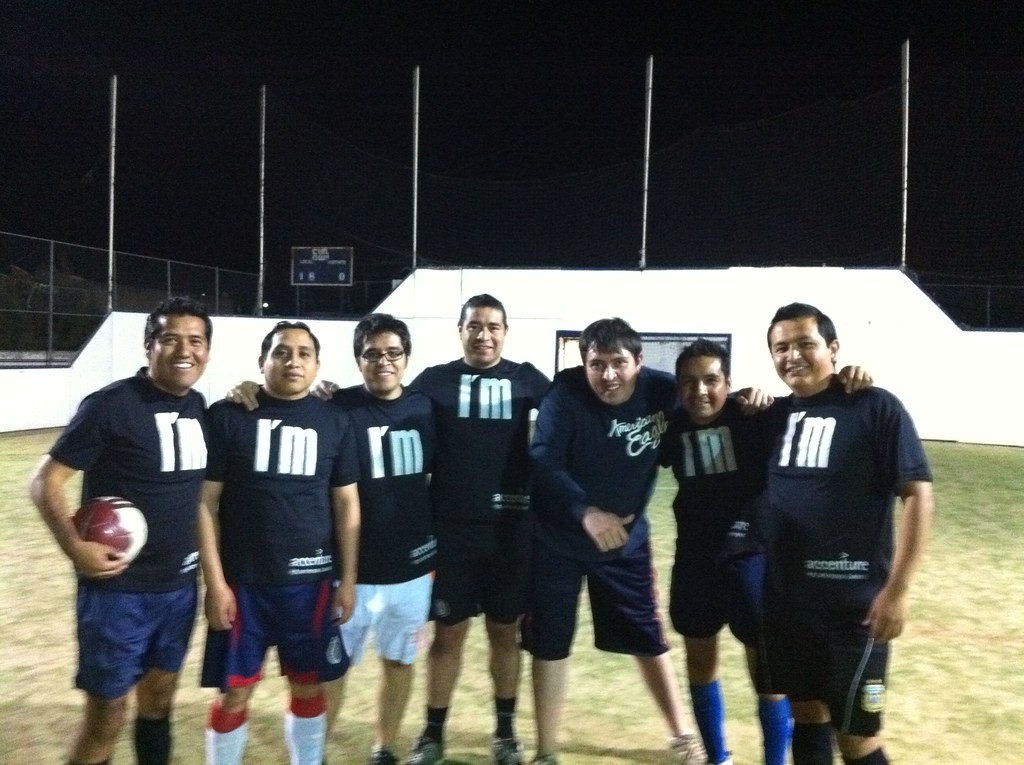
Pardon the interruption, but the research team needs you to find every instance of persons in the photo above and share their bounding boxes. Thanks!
[29,295,935,764]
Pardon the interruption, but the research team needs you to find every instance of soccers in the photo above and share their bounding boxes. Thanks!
[68,493,148,568]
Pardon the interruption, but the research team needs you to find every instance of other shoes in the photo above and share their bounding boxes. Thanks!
[490,731,522,765]
[401,737,445,765]
[366,750,396,765]
[533,753,559,765]
[670,731,708,765]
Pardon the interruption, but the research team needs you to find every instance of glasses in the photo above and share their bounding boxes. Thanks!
[359,348,406,362]
